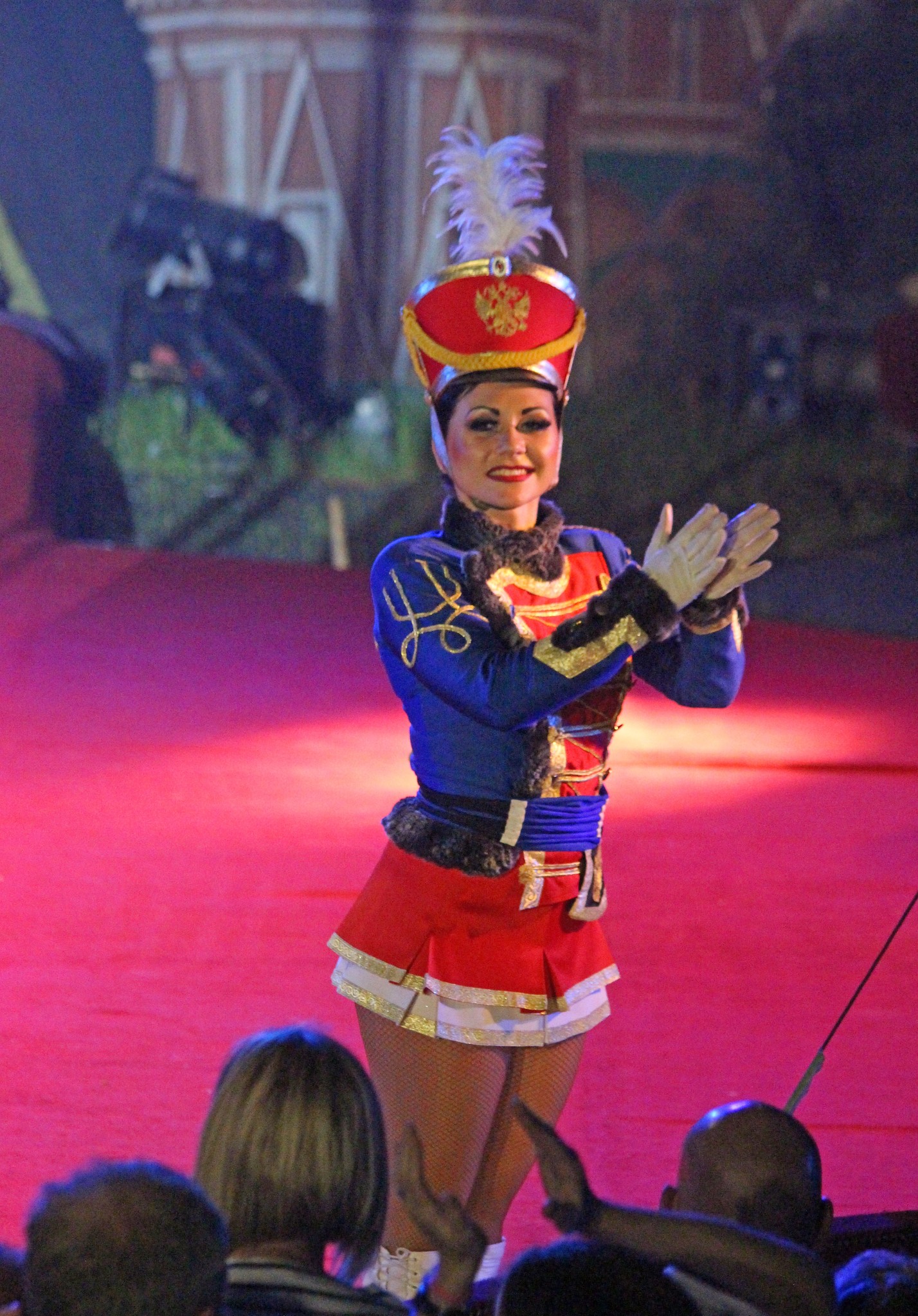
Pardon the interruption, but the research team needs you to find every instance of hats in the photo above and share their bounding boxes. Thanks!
[398,124,587,409]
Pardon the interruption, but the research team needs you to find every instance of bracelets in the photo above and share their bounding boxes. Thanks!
[542,1195,605,1234]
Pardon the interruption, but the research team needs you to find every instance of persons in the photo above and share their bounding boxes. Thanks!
[327,124,784,1307]
[0,1025,918,1316]
